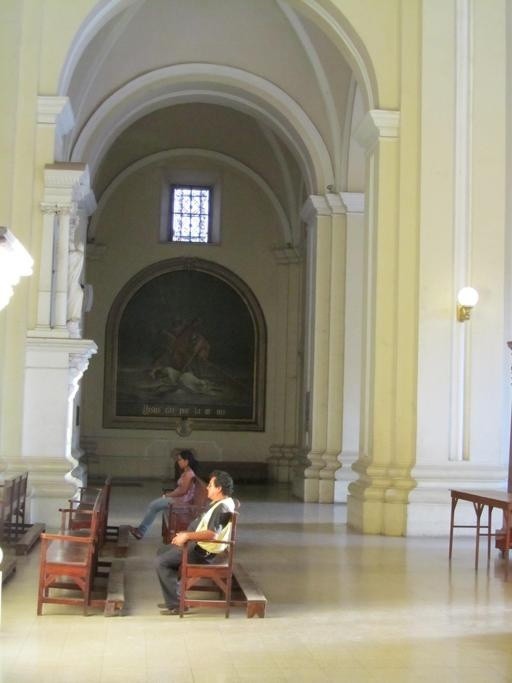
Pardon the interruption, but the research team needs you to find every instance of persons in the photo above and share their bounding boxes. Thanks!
[126,447,198,540]
[152,468,240,615]
[160,315,212,378]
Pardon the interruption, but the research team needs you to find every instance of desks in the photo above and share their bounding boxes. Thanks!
[448,487,512,569]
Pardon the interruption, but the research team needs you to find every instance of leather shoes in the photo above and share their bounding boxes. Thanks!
[156,602,183,614]
[128,524,143,540]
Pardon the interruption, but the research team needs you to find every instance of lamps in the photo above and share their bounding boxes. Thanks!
[454,285,481,322]
